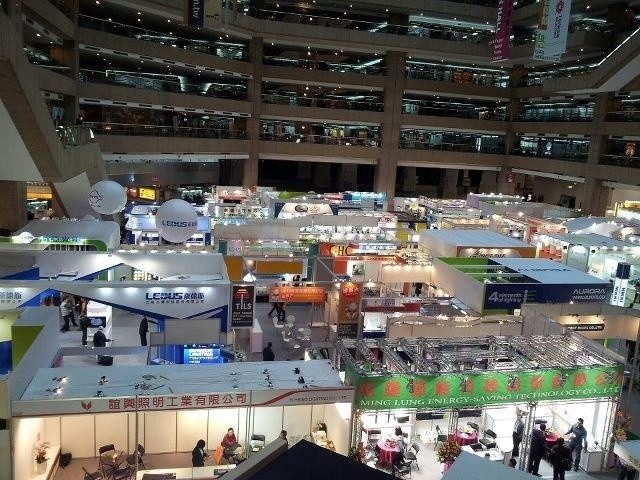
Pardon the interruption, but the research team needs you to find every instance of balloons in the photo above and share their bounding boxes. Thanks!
[154,199,199,244]
[88,180,127,215]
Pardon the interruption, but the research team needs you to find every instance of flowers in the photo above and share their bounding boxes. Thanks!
[33,441,52,464]
[436,441,462,464]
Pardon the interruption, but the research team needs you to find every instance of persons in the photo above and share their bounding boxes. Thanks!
[293,275,300,287]
[60,296,87,345]
[491,76,496,86]
[221,428,236,448]
[265,301,285,321]
[192,439,208,467]
[54,115,62,128]
[617,469,634,480]
[263,341,274,361]
[633,282,640,304]
[93,325,112,362]
[76,113,84,125]
[577,108,580,116]
[139,315,149,346]
[508,413,587,480]
[279,429,288,441]
[391,426,404,476]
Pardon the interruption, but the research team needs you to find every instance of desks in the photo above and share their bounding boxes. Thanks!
[546,432,559,447]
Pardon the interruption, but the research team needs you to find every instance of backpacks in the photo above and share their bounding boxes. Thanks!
[555,445,572,471]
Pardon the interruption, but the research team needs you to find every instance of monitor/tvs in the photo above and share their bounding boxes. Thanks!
[137,186,156,201]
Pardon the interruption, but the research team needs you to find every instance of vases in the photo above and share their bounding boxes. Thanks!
[36,463,47,474]
[443,458,454,474]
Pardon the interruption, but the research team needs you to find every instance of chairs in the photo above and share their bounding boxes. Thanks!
[82,443,146,480]
[534,419,547,429]
[369,441,420,479]
[249,434,265,453]
[433,421,497,452]
